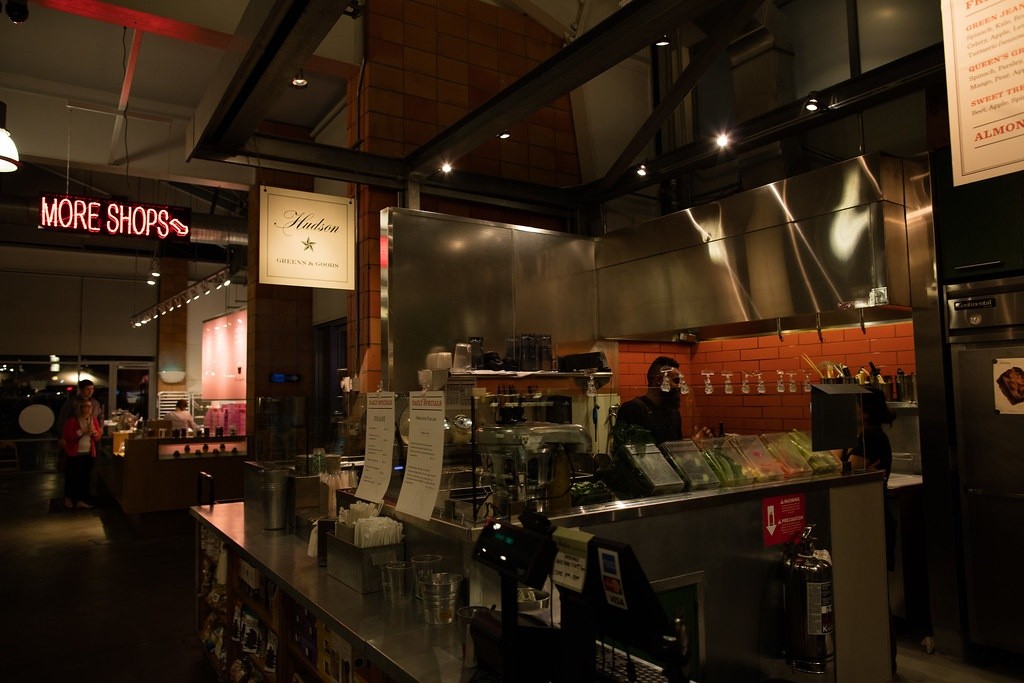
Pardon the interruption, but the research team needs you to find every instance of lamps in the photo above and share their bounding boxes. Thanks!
[130,274,231,328]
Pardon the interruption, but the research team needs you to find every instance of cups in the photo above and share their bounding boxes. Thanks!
[386,562,416,605]
[411,554,441,597]
[428,342,473,373]
[418,570,463,626]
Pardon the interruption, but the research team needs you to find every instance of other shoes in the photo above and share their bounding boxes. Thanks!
[64,498,73,509]
[75,500,93,509]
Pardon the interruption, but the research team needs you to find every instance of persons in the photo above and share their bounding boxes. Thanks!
[165,400,200,434]
[605,356,712,501]
[855,387,899,675]
[57,380,104,508]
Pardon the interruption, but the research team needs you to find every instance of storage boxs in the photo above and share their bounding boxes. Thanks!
[327,536,404,594]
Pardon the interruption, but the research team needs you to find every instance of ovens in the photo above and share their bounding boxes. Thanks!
[942,275,1023,495]
